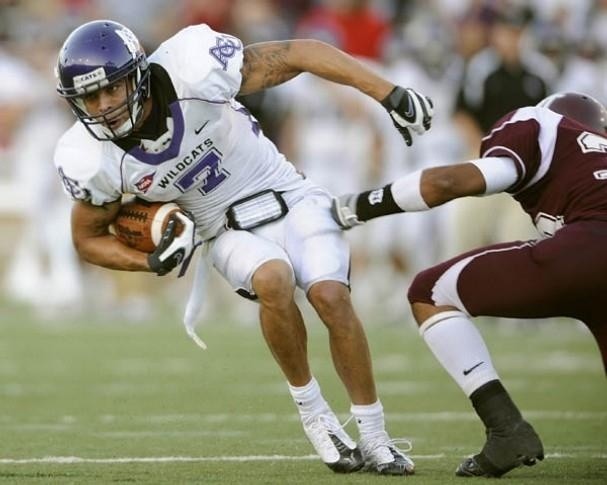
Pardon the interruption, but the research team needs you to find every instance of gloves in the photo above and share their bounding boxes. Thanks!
[148,210,204,278]
[381,86,433,146]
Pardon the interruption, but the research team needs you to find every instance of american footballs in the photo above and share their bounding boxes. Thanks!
[110,198,185,255]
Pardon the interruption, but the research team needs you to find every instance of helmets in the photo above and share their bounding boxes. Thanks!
[535,91,607,135]
[57,20,151,141]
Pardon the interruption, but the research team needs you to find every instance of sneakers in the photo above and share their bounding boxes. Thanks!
[361,431,415,475]
[302,411,363,473]
[455,422,543,477]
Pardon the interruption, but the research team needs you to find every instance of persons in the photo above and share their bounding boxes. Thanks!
[1,0,605,321]
[50,15,438,478]
[322,64,607,485]
